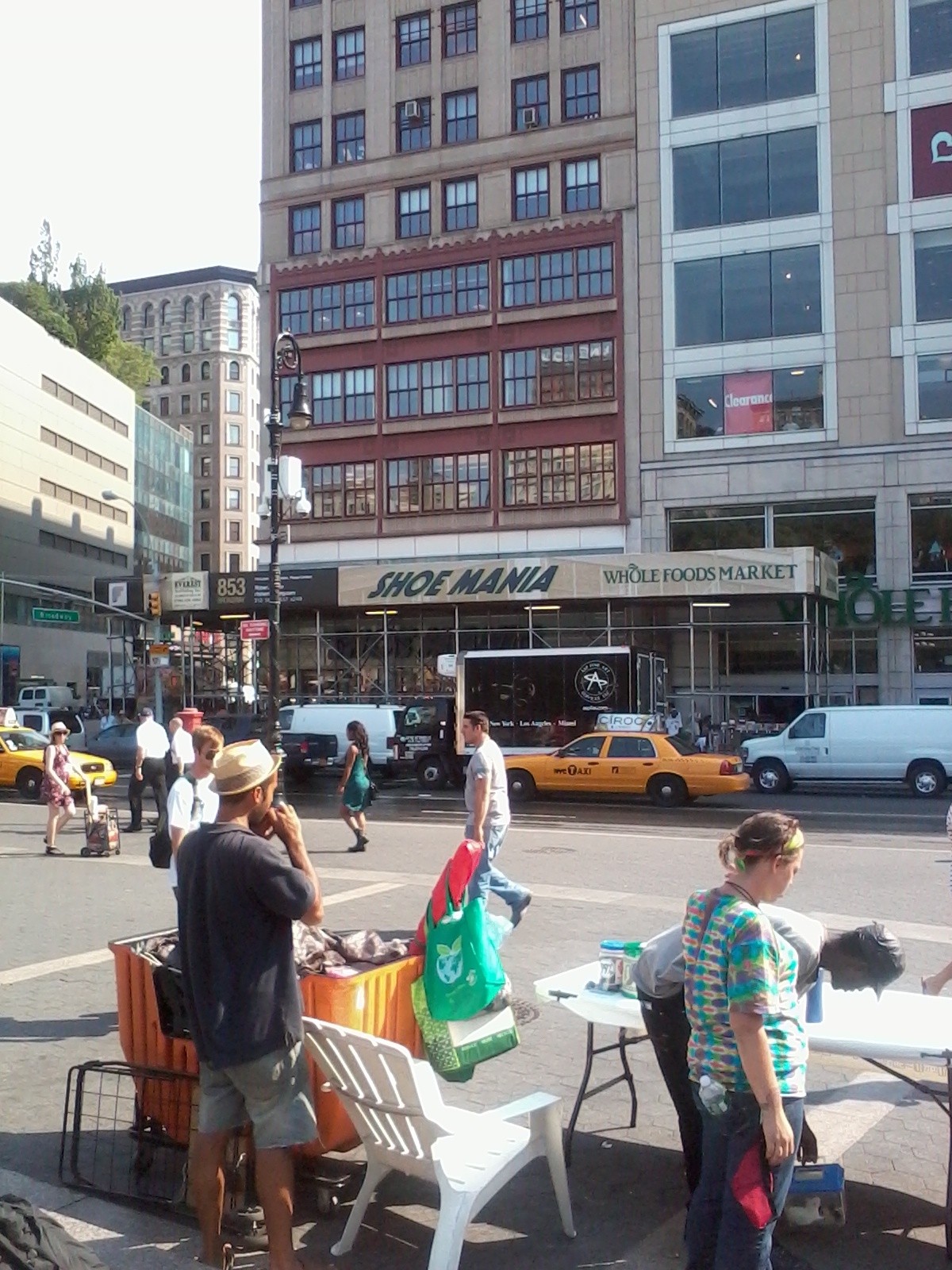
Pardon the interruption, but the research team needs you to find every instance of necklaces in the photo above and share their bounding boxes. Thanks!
[726,880,757,908]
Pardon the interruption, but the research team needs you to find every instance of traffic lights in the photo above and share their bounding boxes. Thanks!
[148,591,161,615]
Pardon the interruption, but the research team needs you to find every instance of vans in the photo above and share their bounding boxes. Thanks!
[738,702,952,799]
[273,703,406,778]
[17,685,80,711]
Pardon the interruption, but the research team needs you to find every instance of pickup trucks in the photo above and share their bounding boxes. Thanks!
[188,714,340,793]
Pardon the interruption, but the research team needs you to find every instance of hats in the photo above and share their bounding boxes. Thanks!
[138,708,154,716]
[857,920,905,1001]
[119,709,125,714]
[49,722,70,737]
[209,739,281,795]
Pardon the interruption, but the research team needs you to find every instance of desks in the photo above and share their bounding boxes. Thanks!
[537,954,952,1264]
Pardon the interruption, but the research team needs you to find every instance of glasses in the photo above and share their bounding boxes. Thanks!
[55,731,68,735]
[202,750,214,761]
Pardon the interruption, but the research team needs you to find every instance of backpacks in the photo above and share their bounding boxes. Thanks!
[149,772,196,869]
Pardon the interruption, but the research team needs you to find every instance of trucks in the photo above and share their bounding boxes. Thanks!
[392,647,674,790]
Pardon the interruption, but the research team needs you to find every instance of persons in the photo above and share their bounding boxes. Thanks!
[40,722,89,857]
[99,708,116,730]
[678,809,811,1270]
[112,708,133,727]
[663,704,723,754]
[334,719,377,855]
[146,717,196,826]
[77,705,86,723]
[457,709,533,928]
[120,707,171,833]
[88,705,99,719]
[921,804,951,997]
[172,738,328,1270]
[630,903,906,1270]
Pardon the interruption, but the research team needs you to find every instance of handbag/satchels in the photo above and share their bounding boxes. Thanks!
[371,782,379,801]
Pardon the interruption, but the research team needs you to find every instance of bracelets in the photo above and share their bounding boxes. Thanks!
[135,765,143,770]
[339,783,346,787]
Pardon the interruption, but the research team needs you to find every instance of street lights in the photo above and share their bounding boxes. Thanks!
[103,490,166,726]
[263,327,314,741]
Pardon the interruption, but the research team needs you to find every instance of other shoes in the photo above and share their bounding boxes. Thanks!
[198,1245,234,1270]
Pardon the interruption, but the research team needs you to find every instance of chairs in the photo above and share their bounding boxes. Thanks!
[293,1016,584,1269]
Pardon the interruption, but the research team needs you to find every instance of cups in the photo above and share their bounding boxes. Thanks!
[621,942,643,999]
[599,939,623,993]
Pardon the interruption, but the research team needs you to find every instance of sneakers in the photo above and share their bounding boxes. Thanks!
[43,817,159,855]
[349,845,365,851]
[354,835,369,848]
[510,890,534,929]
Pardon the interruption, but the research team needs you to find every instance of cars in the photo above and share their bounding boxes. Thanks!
[0,707,118,800]
[503,729,751,809]
[88,722,147,769]
[11,706,89,752]
